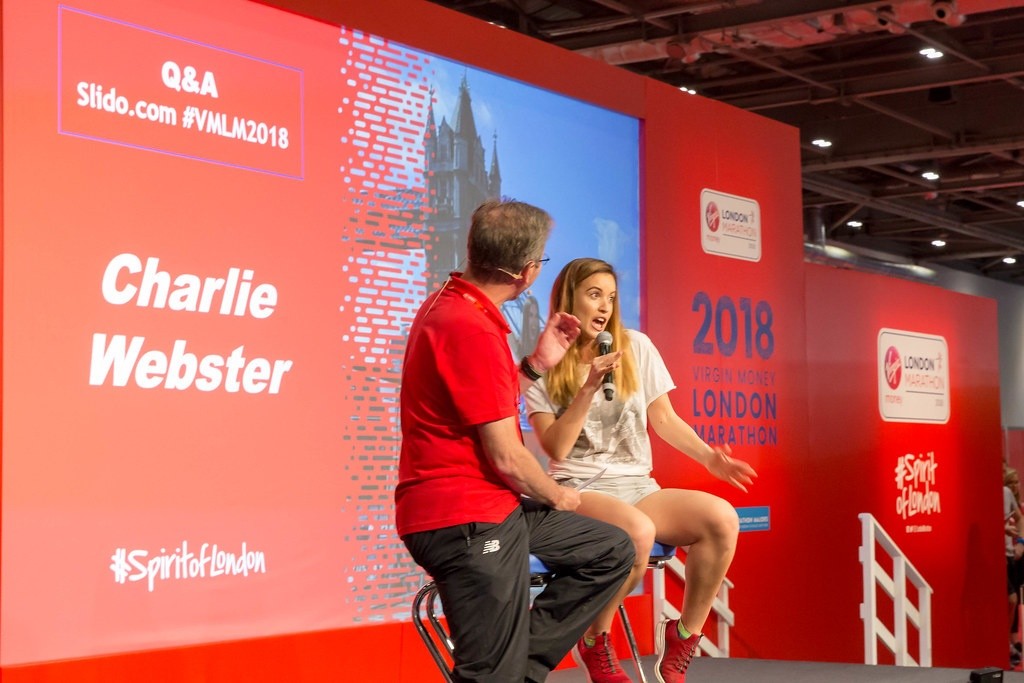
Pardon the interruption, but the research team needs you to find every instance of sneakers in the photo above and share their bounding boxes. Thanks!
[571,631,634,683]
[654,617,704,683]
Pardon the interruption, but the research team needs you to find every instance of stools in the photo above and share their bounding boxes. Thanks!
[409,558,552,683]
[585,542,678,683]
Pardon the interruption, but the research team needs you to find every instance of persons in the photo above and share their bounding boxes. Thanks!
[523,257,758,683]
[394,200,636,683]
[1003,459,1024,665]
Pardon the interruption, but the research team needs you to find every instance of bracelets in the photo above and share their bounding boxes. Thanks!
[520,355,542,381]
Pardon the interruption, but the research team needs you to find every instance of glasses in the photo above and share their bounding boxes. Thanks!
[520,252,550,275]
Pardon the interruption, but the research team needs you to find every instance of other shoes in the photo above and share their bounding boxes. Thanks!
[1010,642,1022,663]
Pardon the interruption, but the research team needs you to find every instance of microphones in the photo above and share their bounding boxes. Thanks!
[597,331,615,401]
